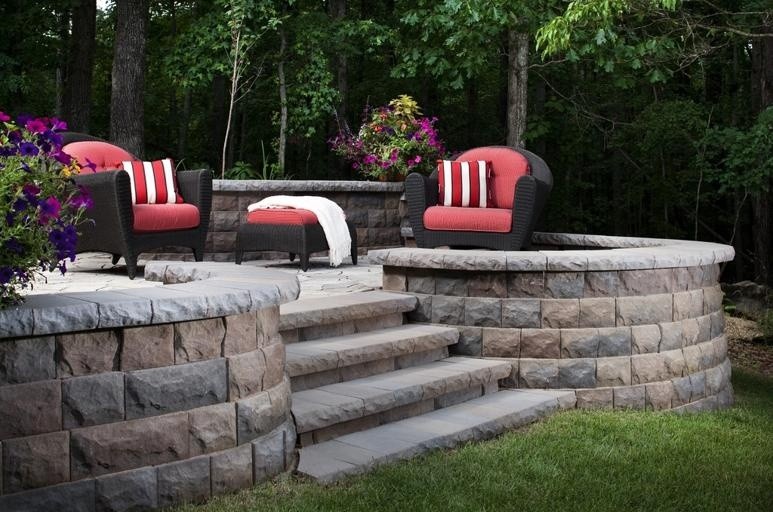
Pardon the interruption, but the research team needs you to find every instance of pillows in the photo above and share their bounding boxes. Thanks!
[62,140,135,174]
[437,159,495,208]
[455,146,530,210]
[120,157,184,204]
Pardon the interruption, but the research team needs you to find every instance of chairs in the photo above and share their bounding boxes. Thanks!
[404,145,554,251]
[55,131,211,278]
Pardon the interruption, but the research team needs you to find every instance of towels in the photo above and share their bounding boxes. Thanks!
[247,194,352,267]
